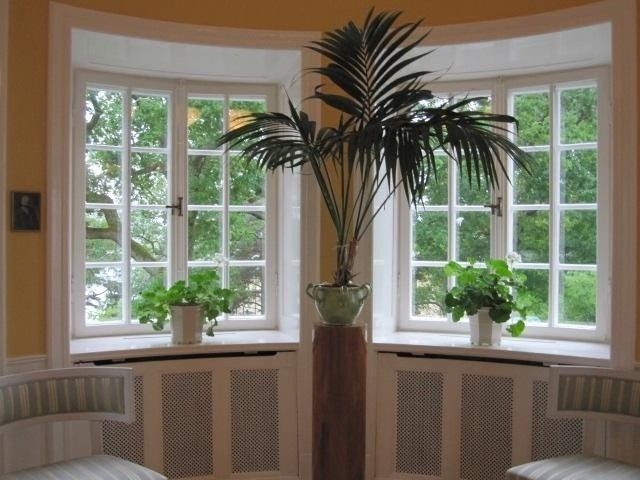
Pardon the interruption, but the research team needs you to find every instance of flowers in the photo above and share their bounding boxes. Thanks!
[441,250,532,337]
[139,252,235,336]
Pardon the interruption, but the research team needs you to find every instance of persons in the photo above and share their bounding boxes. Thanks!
[14,193,40,230]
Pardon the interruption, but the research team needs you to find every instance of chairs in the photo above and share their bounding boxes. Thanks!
[505,363,640,480]
[0,365,168,480]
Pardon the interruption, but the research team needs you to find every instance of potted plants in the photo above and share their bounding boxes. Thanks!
[216,8,540,326]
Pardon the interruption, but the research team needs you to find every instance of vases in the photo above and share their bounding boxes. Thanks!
[467,306,501,346]
[168,304,203,344]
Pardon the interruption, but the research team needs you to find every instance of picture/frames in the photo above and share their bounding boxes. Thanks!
[11,190,42,232]
